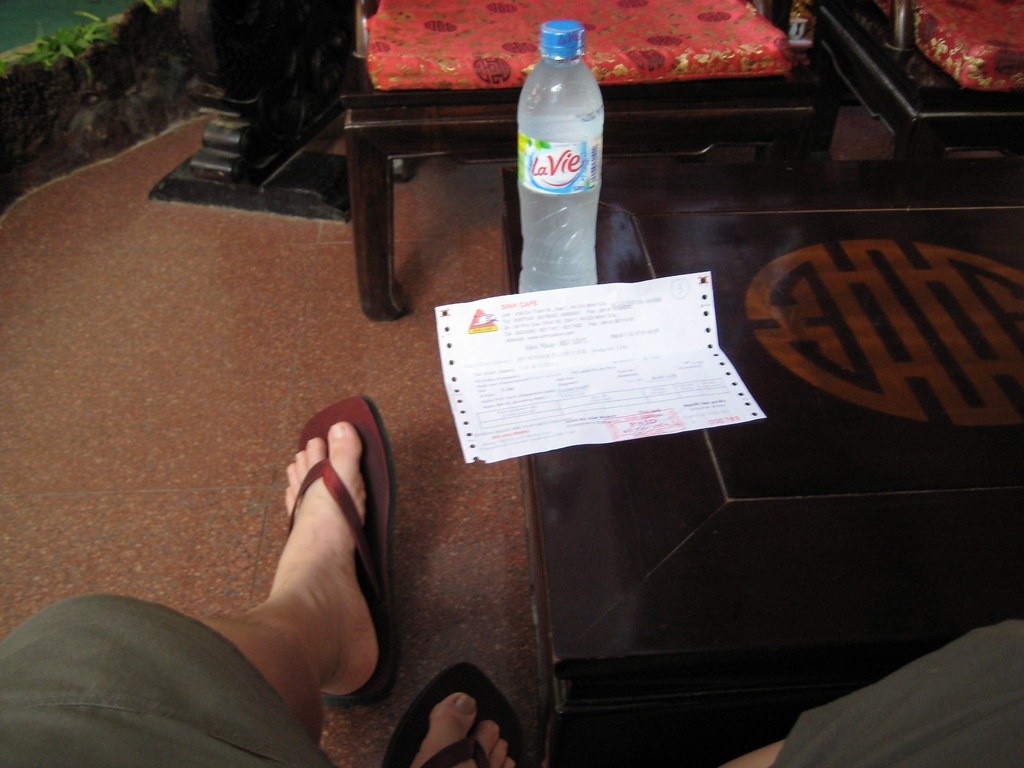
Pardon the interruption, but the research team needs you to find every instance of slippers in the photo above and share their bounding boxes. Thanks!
[382,660,526,768]
[284,398,395,708]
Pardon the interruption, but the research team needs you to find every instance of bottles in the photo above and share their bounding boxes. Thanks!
[513,20,608,294]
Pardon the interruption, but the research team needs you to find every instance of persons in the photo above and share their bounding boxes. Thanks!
[0,396,1024,768]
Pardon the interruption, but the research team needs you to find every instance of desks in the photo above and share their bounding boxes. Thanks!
[501,158,1024,768]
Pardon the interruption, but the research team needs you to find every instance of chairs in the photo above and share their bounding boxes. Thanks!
[805,0,1024,206]
[332,0,837,323]
